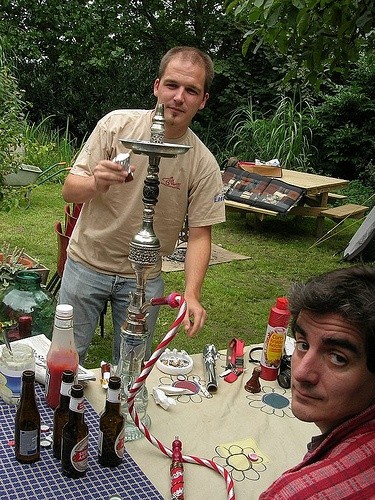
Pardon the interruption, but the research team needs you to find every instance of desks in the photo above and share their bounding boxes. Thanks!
[267,168,350,238]
[0,342,322,500]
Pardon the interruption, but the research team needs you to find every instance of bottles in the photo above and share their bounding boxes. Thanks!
[45,304,80,408]
[260,297,291,382]
[18,317,32,339]
[62,384,89,479]
[98,376,125,468]
[52,370,74,460]
[15,371,41,464]
[0,344,35,406]
[0,271,55,345]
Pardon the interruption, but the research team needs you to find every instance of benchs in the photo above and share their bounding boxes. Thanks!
[225,199,279,216]
[318,192,347,198]
[321,203,369,218]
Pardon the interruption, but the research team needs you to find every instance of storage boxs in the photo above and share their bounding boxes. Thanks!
[0,251,49,284]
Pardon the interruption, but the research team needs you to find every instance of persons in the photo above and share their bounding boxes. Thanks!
[259,265,375,500]
[58,46,226,366]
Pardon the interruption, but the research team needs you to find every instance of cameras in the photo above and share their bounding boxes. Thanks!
[277,355,291,388]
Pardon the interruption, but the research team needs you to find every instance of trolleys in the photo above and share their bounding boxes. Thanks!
[2,161,72,211]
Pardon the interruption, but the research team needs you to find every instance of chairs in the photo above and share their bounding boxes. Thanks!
[45,203,108,338]
